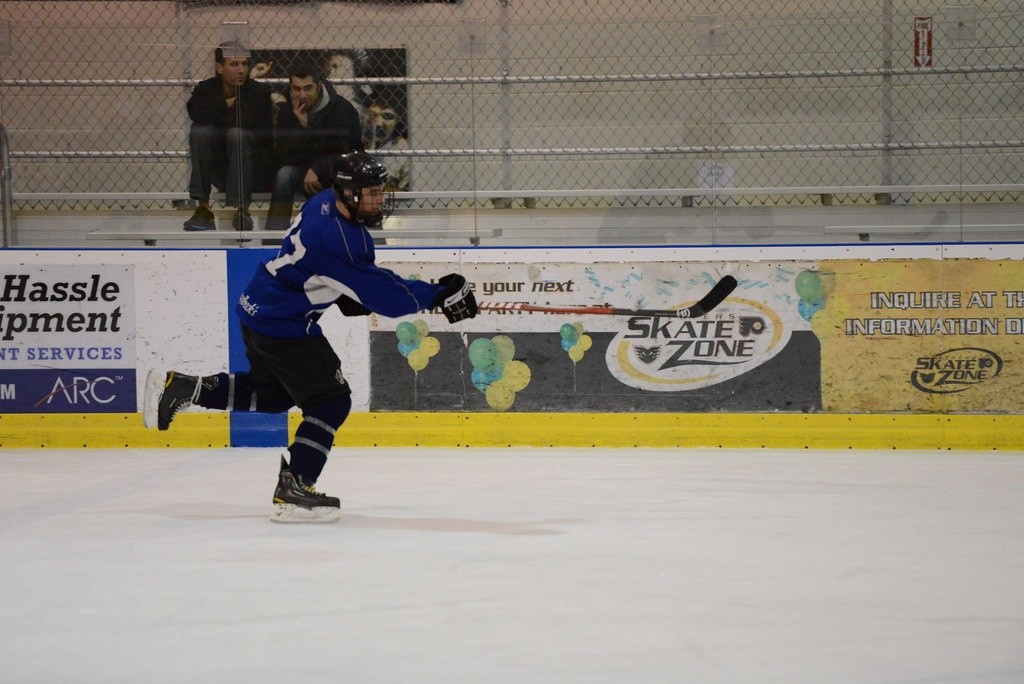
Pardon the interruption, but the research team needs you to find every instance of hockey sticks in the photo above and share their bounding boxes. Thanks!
[475,274,739,319]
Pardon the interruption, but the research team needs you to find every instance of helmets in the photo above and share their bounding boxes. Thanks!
[333,150,398,234]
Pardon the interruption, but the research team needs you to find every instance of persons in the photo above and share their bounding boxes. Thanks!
[183,41,277,242]
[143,151,479,523]
[257,63,387,245]
[247,56,406,192]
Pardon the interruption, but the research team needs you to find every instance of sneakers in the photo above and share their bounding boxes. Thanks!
[143,368,200,430]
[182,205,217,230]
[232,209,255,244]
[272,468,341,524]
